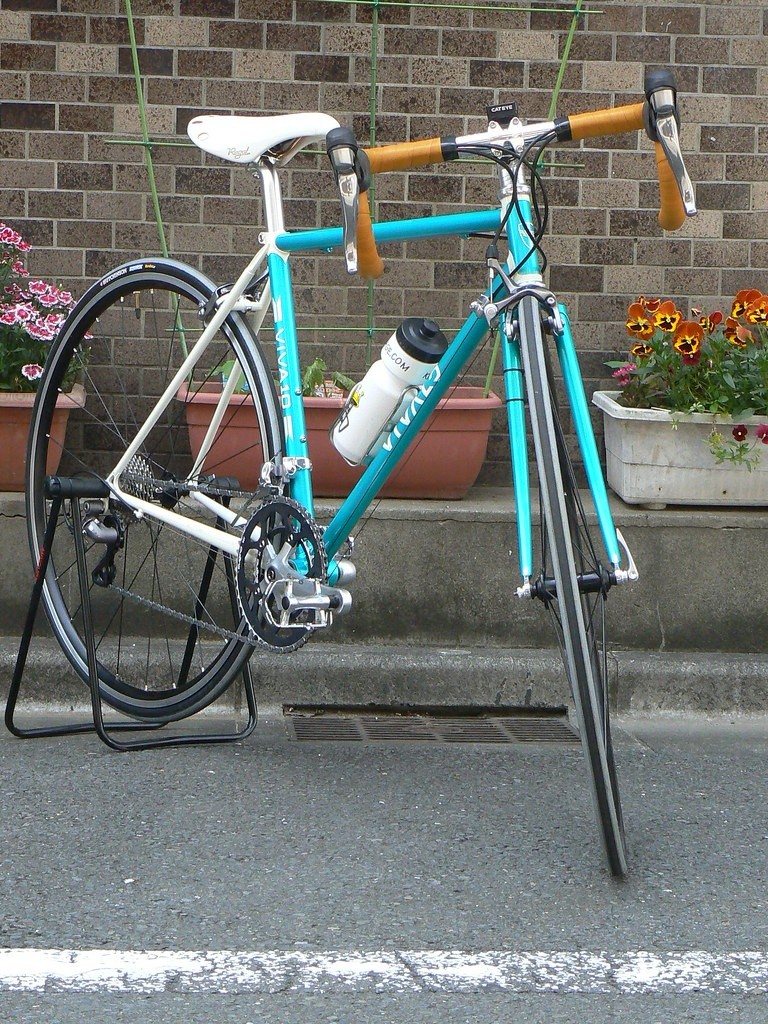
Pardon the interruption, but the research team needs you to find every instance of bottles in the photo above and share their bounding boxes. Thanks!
[330,316,450,463]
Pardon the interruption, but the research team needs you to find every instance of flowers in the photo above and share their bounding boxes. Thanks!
[0,221,95,392]
[603,288,768,473]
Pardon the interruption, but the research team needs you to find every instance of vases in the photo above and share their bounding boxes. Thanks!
[591,390,768,510]
[0,384,87,491]
[177,361,502,500]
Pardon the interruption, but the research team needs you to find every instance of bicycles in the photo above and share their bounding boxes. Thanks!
[25,61,698,876]
